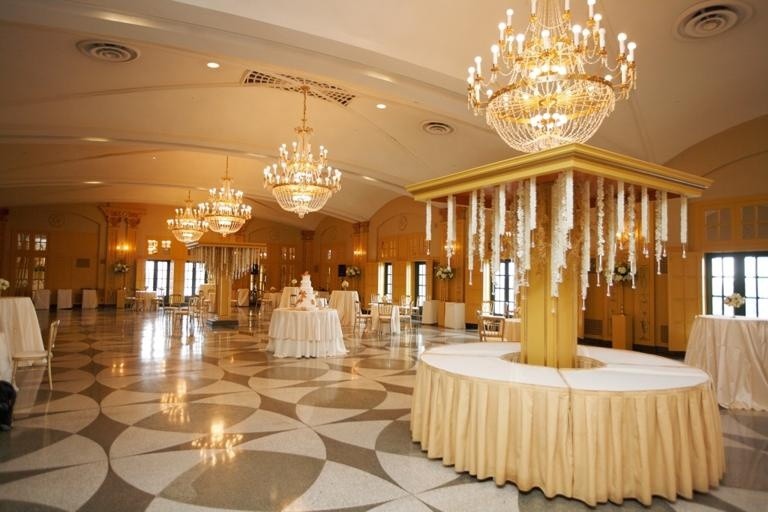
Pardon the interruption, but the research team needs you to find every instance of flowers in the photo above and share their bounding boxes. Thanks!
[345,265,361,277]
[603,261,638,282]
[433,264,455,281]
[113,260,131,273]
[724,292,746,310]
[0,278,10,291]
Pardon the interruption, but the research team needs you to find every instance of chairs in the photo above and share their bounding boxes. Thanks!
[11,319,60,392]
[475,310,505,341]
[123,278,418,358]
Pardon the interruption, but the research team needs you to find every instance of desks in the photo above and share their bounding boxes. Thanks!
[683,314,768,414]
[410,339,726,508]
[0,332,20,394]
[0,296,48,368]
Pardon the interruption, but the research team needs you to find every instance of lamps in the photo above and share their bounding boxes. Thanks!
[198,155,252,238]
[166,189,208,248]
[264,84,341,218]
[111,354,244,466]
[467,0,637,154]
